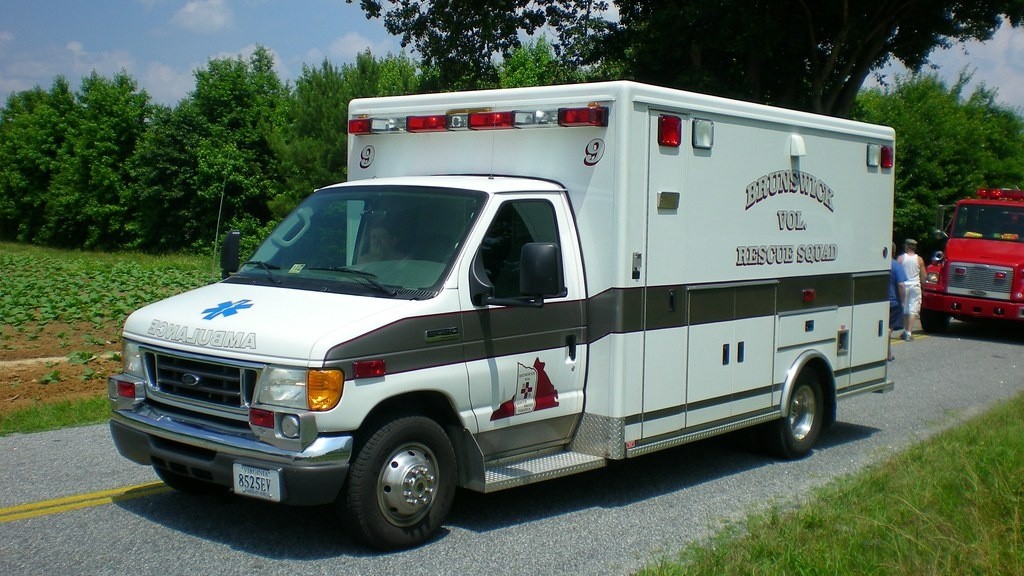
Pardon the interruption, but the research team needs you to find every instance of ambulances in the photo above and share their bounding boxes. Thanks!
[108,81,895,553]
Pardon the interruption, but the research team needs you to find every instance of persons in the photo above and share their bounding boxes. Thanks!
[897,238,928,341]
[355,222,413,263]
[887,241,910,361]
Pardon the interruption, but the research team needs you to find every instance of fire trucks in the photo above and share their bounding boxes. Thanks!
[920,188,1024,333]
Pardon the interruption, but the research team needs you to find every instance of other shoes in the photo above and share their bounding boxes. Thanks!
[900,331,912,341]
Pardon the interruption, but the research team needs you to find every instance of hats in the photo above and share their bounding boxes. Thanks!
[903,238,919,249]
[931,251,943,261]
